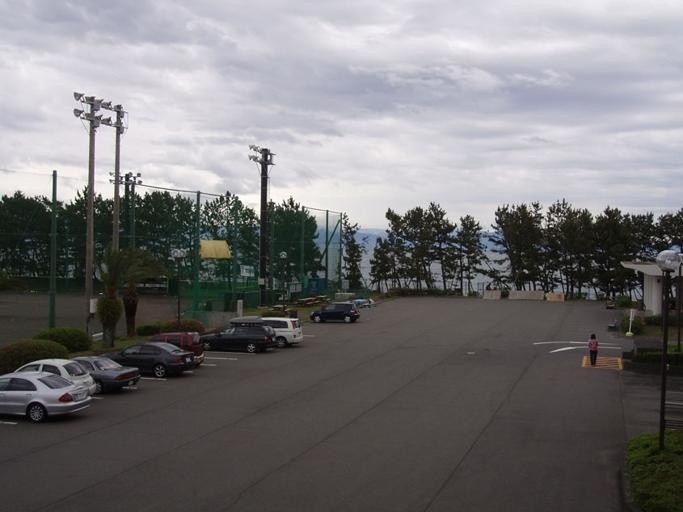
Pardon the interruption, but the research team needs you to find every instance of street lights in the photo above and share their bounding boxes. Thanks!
[655,249,681,448]
[279,251,287,312]
[171,248,186,321]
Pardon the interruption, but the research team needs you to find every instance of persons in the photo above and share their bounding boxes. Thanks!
[588,334,599,366]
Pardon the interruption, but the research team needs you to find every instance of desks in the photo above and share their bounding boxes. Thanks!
[297,295,328,308]
[272,305,287,311]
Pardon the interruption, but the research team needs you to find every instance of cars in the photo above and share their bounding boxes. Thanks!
[71,355,140,395]
[13,359,96,396]
[150,331,204,369]
[99,341,194,378]
[0,371,92,423]
[208,326,278,353]
[260,317,303,348]
[309,302,359,323]
